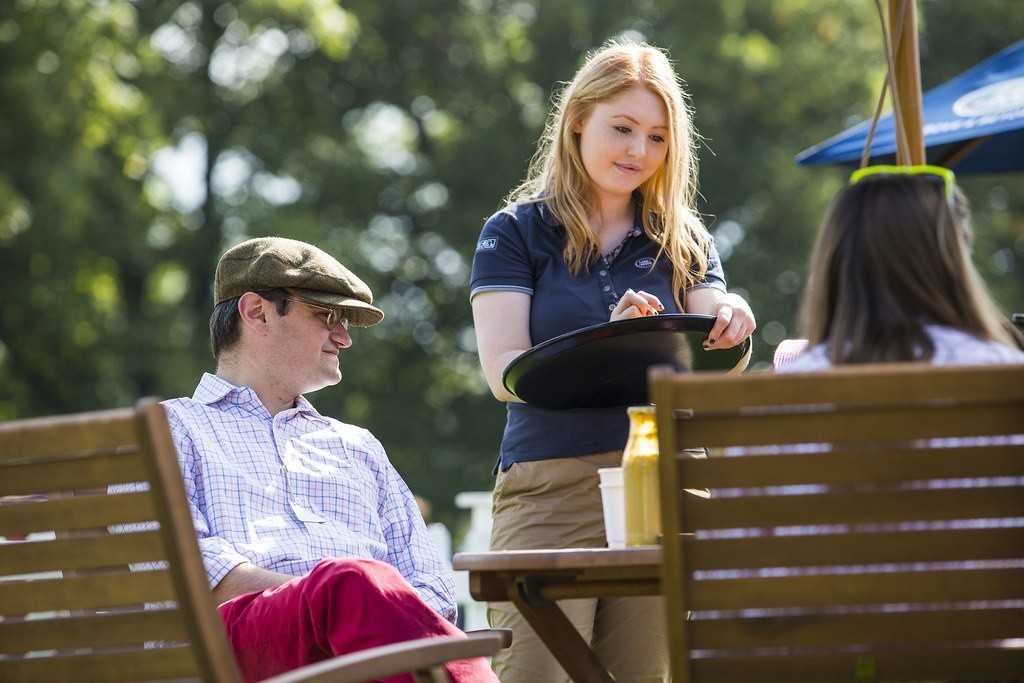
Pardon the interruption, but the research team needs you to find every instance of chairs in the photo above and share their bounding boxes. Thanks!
[0,393,502,683]
[651,365,1024,683]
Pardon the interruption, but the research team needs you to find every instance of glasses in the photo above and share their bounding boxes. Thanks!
[846,160,960,200]
[279,296,351,329]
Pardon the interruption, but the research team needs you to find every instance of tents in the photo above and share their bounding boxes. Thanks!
[793,39,1024,175]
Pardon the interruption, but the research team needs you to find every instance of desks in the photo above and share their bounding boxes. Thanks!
[447,546,669,683]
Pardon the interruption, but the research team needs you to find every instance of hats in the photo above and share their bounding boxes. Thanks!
[212,236,385,328]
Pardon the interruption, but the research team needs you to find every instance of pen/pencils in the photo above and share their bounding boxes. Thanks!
[626,285,661,318]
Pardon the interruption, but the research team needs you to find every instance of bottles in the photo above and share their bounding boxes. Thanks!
[624,406,667,546]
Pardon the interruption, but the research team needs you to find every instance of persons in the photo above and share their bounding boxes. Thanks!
[689,164,1024,662]
[106,237,502,682]
[468,36,756,682]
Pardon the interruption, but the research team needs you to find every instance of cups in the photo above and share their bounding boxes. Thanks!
[597,468,623,483]
[598,484,625,549]
